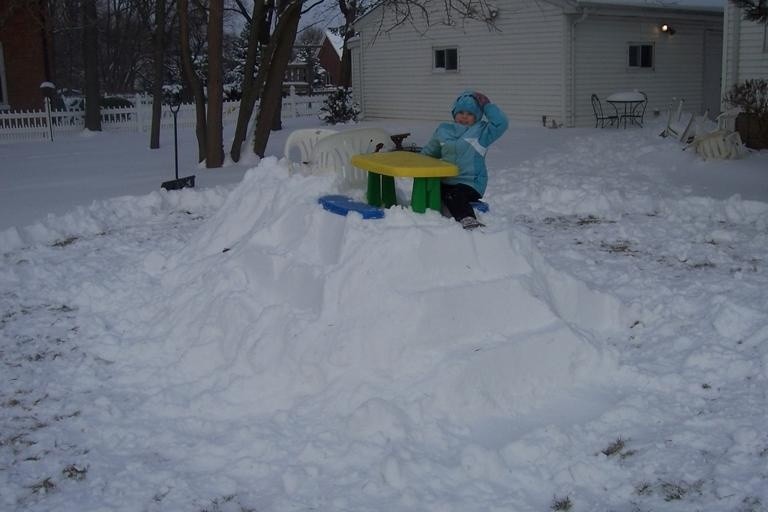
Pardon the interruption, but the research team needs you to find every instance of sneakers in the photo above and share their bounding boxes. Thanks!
[458,216,486,230]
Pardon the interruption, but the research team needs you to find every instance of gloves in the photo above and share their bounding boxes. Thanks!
[474,91,491,107]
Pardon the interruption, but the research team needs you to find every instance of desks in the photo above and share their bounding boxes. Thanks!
[606,99,645,129]
[351,149,461,215]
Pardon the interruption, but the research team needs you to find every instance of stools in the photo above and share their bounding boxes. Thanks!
[319,194,385,220]
[470,198,491,215]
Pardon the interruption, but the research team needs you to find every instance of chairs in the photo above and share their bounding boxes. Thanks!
[281,127,338,169]
[626,91,649,126]
[658,98,744,166]
[590,93,620,127]
[308,127,394,185]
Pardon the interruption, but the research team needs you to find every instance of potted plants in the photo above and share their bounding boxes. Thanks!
[722,79,768,152]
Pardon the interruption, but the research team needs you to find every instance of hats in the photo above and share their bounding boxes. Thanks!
[450,89,484,123]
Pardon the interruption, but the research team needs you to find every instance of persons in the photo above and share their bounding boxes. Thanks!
[419,91,509,231]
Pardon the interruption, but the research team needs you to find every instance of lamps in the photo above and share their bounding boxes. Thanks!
[660,23,676,36]
